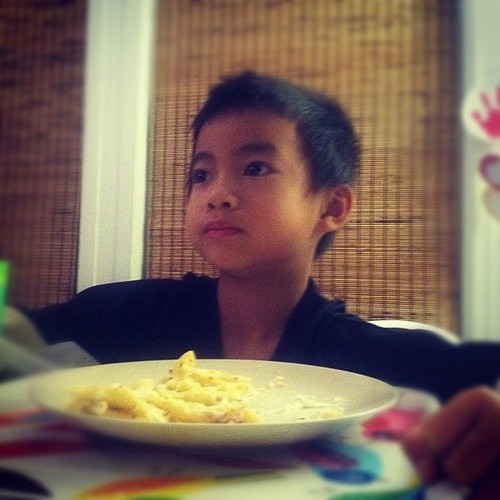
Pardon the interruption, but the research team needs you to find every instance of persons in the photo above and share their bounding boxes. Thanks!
[29,70,500,490]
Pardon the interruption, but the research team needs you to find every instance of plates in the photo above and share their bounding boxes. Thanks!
[33,358,397,449]
